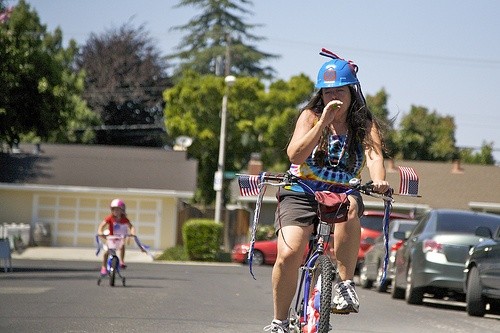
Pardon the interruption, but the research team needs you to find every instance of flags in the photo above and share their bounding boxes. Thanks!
[238,175,259,196]
[399,166,420,195]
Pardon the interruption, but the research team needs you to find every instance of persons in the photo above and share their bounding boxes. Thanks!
[97,198,136,277]
[263,49,389,333]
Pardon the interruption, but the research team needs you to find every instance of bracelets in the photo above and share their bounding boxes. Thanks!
[318,120,326,129]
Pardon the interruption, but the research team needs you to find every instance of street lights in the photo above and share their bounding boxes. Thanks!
[213,73,237,224]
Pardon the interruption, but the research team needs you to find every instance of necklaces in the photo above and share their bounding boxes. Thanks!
[328,129,348,167]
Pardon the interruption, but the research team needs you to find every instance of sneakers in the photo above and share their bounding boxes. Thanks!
[263,318,291,333]
[332,279,360,314]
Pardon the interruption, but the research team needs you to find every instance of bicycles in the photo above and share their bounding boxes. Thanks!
[237,171,396,332]
[95,233,131,287]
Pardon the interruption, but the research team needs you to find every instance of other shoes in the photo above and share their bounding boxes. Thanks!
[100,268,108,276]
[119,263,127,270]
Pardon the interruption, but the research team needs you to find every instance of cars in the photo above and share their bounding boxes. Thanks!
[393,209,499,305]
[328,211,408,270]
[461,222,499,315]
[358,219,419,293]
[231,224,328,265]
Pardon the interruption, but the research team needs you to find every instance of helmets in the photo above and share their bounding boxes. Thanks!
[315,58,360,88]
[110,198,125,211]
